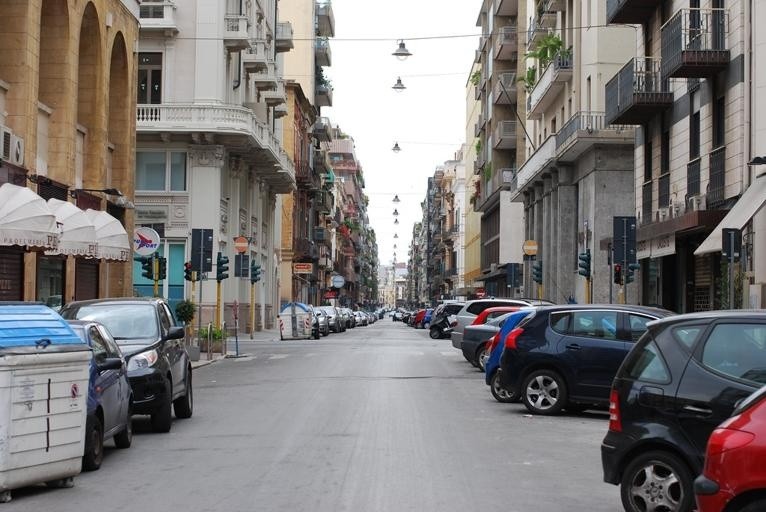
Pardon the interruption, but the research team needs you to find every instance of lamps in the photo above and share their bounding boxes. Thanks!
[391,40,413,238]
[71,187,125,200]
[13,172,53,187]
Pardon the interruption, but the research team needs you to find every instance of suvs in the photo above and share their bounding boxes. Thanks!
[62,296,194,433]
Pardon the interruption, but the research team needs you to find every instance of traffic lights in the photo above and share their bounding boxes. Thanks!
[159,258,166,280]
[251,260,261,284]
[217,251,230,282]
[194,271,200,282]
[184,261,193,282]
[533,262,543,283]
[614,265,622,283]
[142,257,153,280]
[627,264,640,283]
[579,254,591,278]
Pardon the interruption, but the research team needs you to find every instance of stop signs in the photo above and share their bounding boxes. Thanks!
[234,237,248,252]
[522,239,538,255]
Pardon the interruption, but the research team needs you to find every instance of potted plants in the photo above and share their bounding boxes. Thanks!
[522,32,564,59]
[198,322,230,353]
[175,298,200,361]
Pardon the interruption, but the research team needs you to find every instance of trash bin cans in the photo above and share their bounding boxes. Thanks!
[1,300,94,504]
[280,302,313,339]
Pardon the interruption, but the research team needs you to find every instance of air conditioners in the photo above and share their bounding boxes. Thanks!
[668,205,680,220]
[0,123,12,163]
[12,135,24,166]
[654,210,666,223]
[688,195,707,212]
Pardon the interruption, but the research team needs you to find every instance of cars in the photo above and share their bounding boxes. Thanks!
[64,317,133,469]
[46,294,63,313]
[303,304,392,340]
